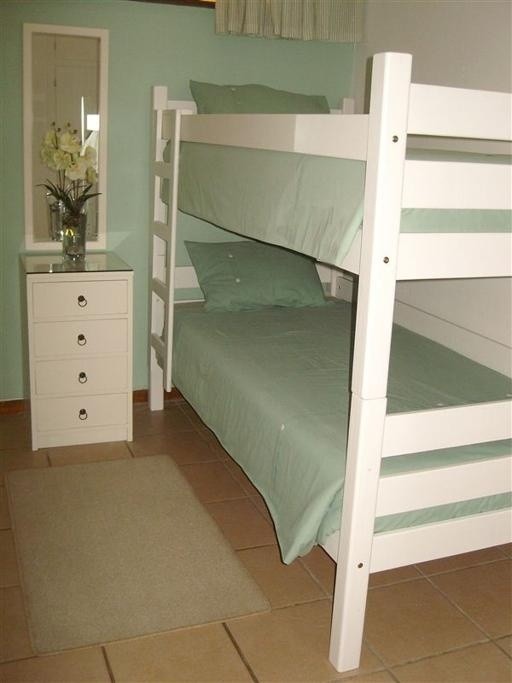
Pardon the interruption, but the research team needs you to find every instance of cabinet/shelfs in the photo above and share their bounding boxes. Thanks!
[18,256,137,453]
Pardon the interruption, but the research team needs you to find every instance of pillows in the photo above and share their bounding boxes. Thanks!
[185,231,331,308]
[191,72,334,118]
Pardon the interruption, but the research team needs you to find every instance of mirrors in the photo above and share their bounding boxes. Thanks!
[23,22,109,255]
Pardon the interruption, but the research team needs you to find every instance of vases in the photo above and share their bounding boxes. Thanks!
[62,213,88,264]
[49,204,66,241]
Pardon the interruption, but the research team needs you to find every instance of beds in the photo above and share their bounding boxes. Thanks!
[148,49,511,671]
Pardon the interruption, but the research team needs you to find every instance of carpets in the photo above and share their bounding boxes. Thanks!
[4,453,274,655]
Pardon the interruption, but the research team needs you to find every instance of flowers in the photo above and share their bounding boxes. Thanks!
[35,121,77,229]
[32,115,116,213]
